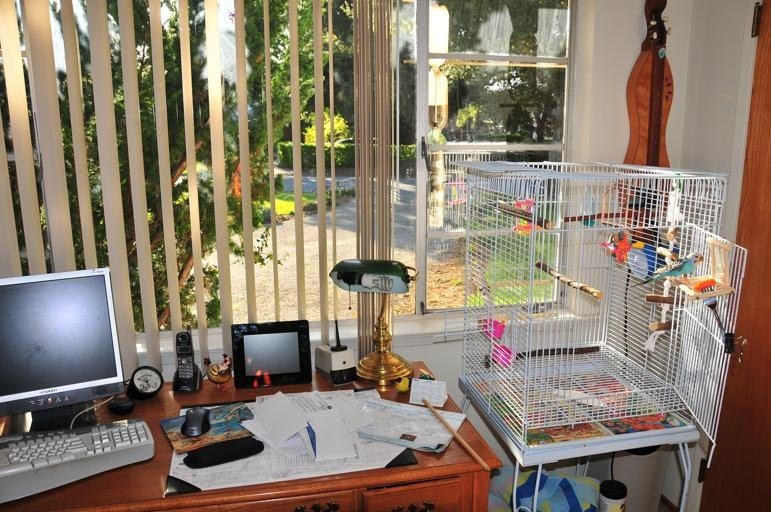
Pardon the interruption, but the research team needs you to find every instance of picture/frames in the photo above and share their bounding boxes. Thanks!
[231,320,311,389]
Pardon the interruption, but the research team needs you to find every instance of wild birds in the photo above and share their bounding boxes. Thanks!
[629,252,704,289]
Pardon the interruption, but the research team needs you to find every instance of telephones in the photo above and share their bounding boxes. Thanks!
[172,325,202,392]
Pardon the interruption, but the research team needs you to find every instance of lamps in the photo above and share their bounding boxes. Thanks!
[331,259,419,385]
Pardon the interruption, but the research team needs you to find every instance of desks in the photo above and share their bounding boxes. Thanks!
[0,362,502,512]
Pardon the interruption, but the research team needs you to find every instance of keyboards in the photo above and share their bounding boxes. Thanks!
[0,418,155,505]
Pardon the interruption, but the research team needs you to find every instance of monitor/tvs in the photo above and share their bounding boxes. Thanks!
[0,267,126,436]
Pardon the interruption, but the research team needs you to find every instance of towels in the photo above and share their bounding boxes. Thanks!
[490,463,603,512]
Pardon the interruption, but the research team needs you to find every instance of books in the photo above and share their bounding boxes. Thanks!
[353,396,466,454]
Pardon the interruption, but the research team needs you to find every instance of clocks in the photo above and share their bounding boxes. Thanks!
[127,365,163,399]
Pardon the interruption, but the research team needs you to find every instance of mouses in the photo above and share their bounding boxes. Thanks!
[181,407,210,437]
